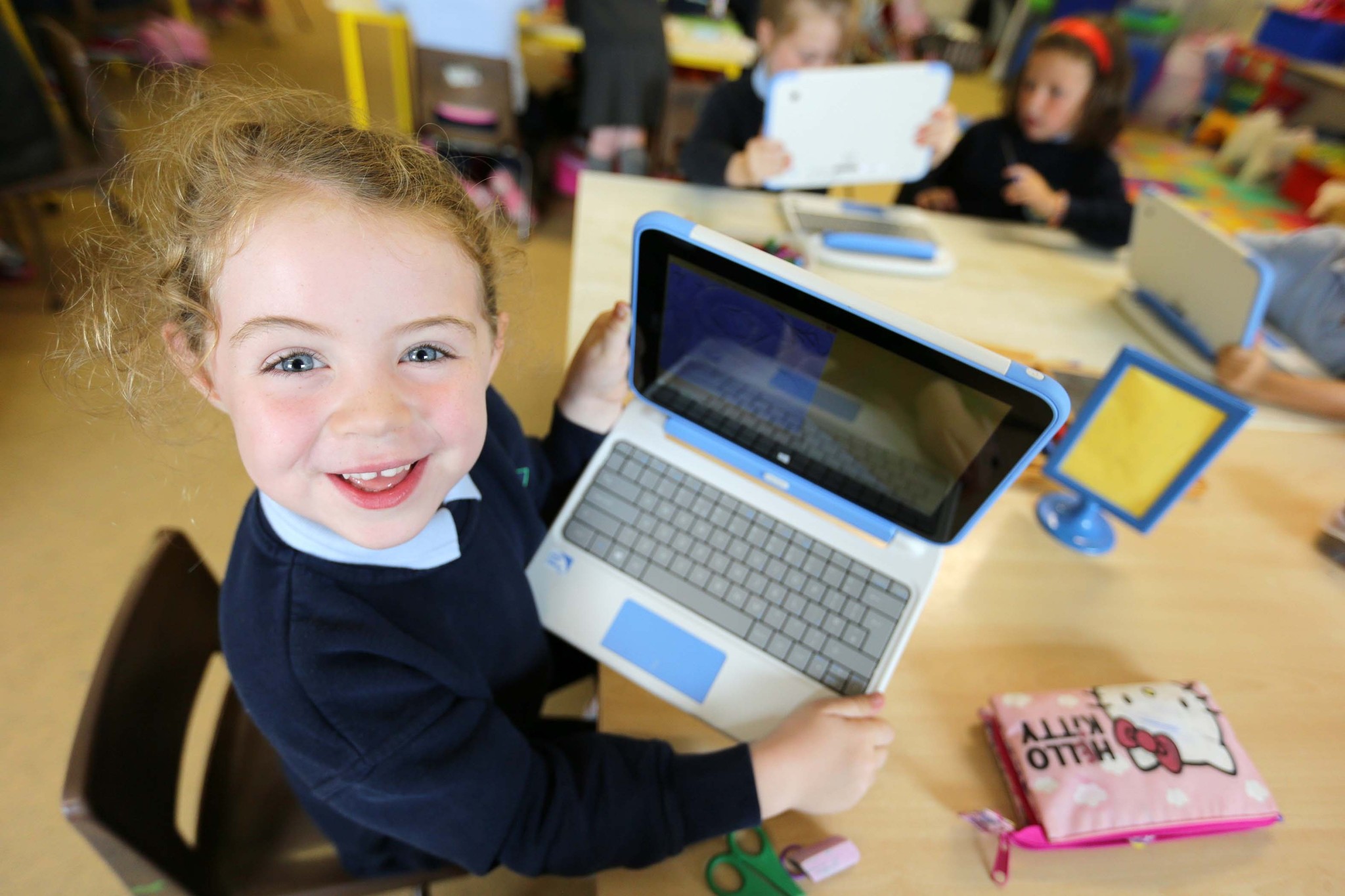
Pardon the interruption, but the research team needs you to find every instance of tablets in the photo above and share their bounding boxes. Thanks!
[779,193,953,278]
[763,58,953,191]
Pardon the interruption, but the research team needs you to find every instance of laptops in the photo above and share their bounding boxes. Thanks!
[1123,185,1304,366]
[522,212,1074,744]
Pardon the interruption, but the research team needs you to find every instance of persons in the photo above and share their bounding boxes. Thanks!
[896,12,1135,252]
[890,361,988,482]
[1214,224,1345,422]
[67,82,893,880]
[520,0,960,203]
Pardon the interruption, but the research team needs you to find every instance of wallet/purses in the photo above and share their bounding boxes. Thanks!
[966,682,1283,884]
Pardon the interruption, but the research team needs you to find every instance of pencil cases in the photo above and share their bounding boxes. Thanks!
[958,678,1284,886]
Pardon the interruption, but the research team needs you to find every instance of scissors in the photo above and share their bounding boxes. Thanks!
[705,825,804,896]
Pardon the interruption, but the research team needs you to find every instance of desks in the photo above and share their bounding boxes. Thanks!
[547,169,1343,896]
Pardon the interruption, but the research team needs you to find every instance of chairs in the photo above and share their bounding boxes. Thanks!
[417,46,554,224]
[5,11,160,313]
[60,519,462,896]
